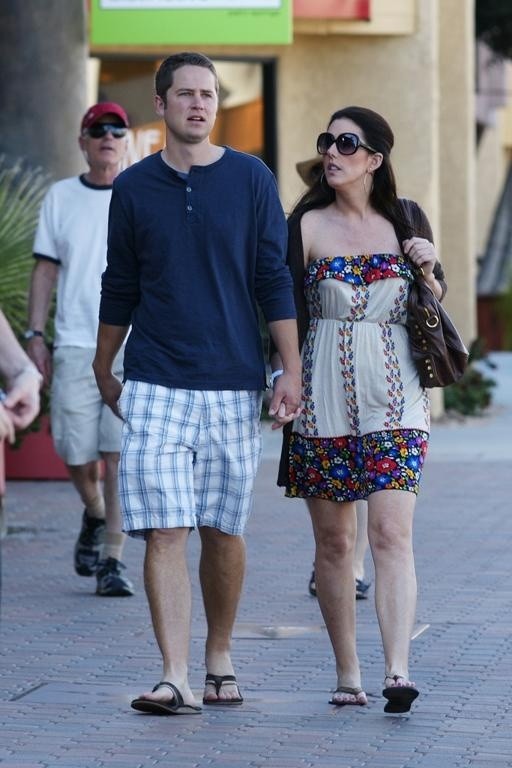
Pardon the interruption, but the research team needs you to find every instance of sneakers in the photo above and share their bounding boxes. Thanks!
[96,555,134,596]
[73,506,107,580]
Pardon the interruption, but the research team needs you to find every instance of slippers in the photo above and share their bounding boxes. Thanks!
[130,683,203,717]
[327,685,367,706]
[381,672,420,714]
[201,672,246,706]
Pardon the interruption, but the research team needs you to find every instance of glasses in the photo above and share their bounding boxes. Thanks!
[315,131,379,156]
[88,123,128,138]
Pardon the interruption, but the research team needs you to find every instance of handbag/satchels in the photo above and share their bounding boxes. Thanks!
[406,277,471,387]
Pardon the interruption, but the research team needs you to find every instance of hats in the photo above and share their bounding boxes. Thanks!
[79,101,130,135]
[295,150,324,189]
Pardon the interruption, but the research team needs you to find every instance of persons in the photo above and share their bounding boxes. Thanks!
[90,50,306,717]
[0,311,41,444]
[264,105,446,714]
[29,101,137,598]
[295,154,368,603]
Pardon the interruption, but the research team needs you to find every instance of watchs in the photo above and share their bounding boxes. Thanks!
[268,369,284,388]
[24,330,41,339]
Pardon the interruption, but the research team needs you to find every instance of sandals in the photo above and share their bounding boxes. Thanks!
[307,560,369,599]
